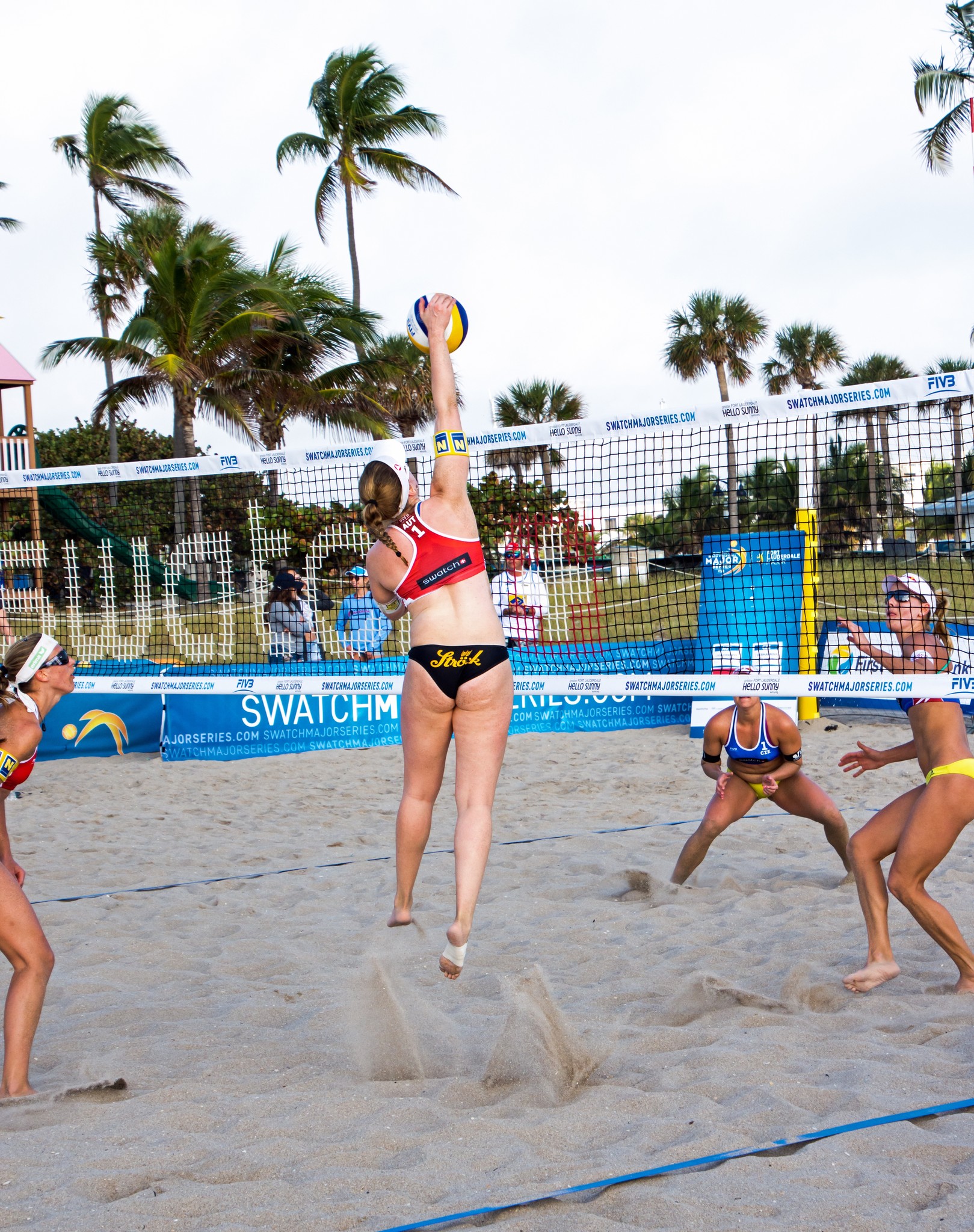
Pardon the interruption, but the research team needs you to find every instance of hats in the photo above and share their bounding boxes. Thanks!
[504,542,525,554]
[881,573,936,617]
[366,439,409,519]
[732,665,758,675]
[273,573,304,591]
[345,566,369,577]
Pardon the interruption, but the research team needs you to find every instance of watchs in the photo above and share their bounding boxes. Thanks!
[525,607,529,615]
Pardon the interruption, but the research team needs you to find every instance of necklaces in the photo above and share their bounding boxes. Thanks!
[28,695,46,731]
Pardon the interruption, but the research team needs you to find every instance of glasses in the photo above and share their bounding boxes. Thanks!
[349,575,359,581]
[38,649,69,670]
[885,590,926,605]
[507,553,520,558]
[404,463,410,480]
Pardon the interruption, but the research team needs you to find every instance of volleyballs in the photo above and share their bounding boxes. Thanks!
[406,293,469,356]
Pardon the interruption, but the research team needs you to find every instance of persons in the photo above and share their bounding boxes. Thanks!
[837,573,974,994]
[490,543,549,648]
[357,290,514,982]
[0,590,17,645]
[335,564,393,662]
[663,664,853,890]
[0,632,76,1100]
[262,566,334,664]
